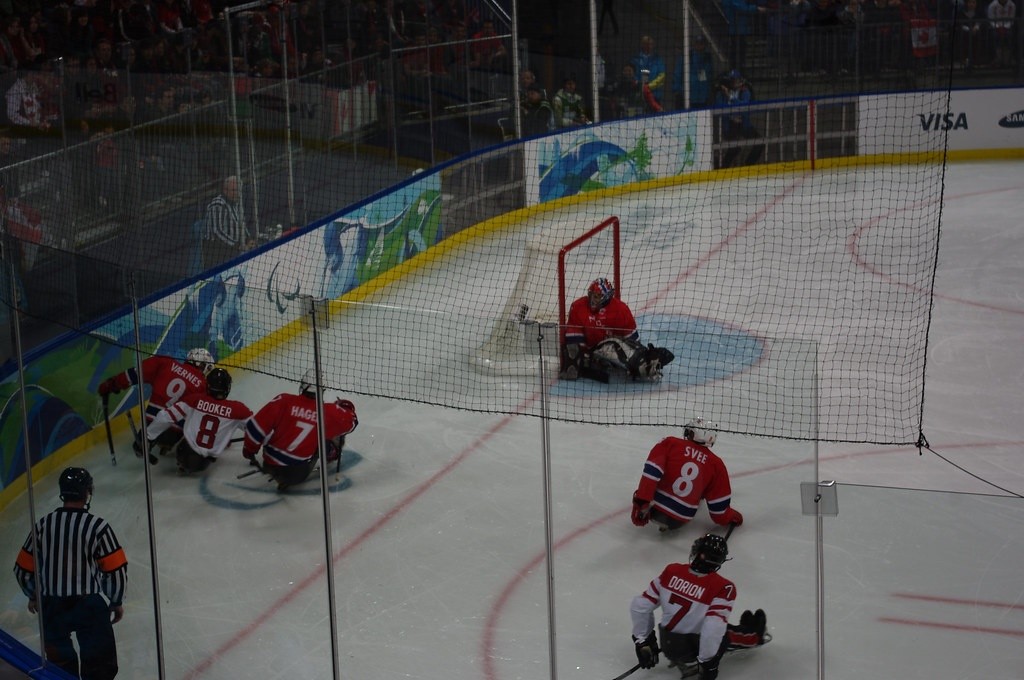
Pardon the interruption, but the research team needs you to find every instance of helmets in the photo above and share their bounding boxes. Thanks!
[588,278,613,310]
[301,368,327,392]
[691,534,728,567]
[59,467,93,495]
[207,367,232,391]
[186,348,215,363]
[685,417,717,443]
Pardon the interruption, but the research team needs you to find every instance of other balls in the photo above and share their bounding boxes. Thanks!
[519,304,528,320]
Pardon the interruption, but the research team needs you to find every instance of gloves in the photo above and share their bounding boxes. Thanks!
[133,429,156,457]
[335,399,355,411]
[696,655,719,680]
[729,511,743,527]
[631,489,650,526]
[632,630,659,669]
[99,376,121,395]
[243,446,253,459]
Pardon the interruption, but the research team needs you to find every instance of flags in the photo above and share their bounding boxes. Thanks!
[0,185,44,274]
[910,19,937,57]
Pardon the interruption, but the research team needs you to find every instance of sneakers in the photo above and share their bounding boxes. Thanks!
[748,609,767,645]
[740,609,752,628]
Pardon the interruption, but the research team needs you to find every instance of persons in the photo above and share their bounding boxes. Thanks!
[631,418,743,531]
[672,33,713,111]
[986,0,1016,65]
[631,533,766,680]
[598,0,619,35]
[588,38,615,124]
[562,278,663,381]
[620,35,666,114]
[12,466,127,680]
[723,0,767,65]
[553,78,588,127]
[766,0,988,68]
[717,69,765,167]
[146,368,254,473]
[518,67,555,136]
[98,348,214,452]
[0,0,510,230]
[243,369,356,483]
[200,176,254,269]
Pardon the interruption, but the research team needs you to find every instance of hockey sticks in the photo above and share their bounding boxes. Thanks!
[102,394,117,466]
[125,408,159,466]
[725,523,736,540]
[230,437,246,442]
[614,648,662,680]
